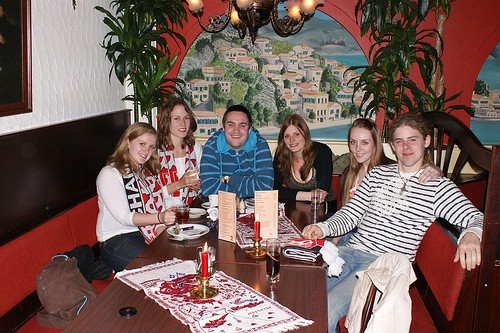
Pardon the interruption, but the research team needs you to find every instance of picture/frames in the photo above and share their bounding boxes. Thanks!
[0,0,33,118]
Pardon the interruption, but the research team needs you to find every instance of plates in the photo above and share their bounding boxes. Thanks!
[201,201,209,208]
[189,207,207,219]
[167,224,210,239]
[245,198,255,208]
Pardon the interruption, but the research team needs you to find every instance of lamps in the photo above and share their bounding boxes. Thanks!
[187,0,324,45]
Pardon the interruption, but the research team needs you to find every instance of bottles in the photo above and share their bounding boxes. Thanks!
[236,196,240,211]
[239,198,245,213]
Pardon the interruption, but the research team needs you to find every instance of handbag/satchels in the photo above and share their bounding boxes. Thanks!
[35,255,96,319]
[89,244,114,281]
[55,243,95,284]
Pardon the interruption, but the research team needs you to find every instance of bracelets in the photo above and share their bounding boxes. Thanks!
[158,213,162,223]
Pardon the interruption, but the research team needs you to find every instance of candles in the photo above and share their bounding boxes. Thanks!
[255,213,261,239]
[201,242,209,278]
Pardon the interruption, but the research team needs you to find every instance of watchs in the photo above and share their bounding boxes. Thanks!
[224,176,229,184]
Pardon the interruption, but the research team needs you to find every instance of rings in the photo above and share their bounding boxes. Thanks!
[428,170,431,173]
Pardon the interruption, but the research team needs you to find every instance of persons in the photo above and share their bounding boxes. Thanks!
[95,122,176,272]
[273,114,333,203]
[156,98,202,207]
[324,118,440,245]
[199,105,274,198]
[302,113,485,333]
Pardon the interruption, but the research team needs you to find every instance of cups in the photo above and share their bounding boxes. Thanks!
[172,204,190,224]
[310,181,320,210]
[209,195,218,207]
[197,246,216,275]
[265,238,280,283]
[206,208,219,230]
[186,167,199,187]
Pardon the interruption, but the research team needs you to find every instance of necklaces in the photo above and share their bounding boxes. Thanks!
[398,168,421,194]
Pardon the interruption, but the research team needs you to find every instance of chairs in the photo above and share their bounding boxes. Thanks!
[359,283,383,333]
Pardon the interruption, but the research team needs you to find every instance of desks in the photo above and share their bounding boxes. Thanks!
[63,258,331,333]
[138,193,325,268]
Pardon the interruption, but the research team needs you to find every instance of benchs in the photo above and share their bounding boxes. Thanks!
[0,111,499,333]
